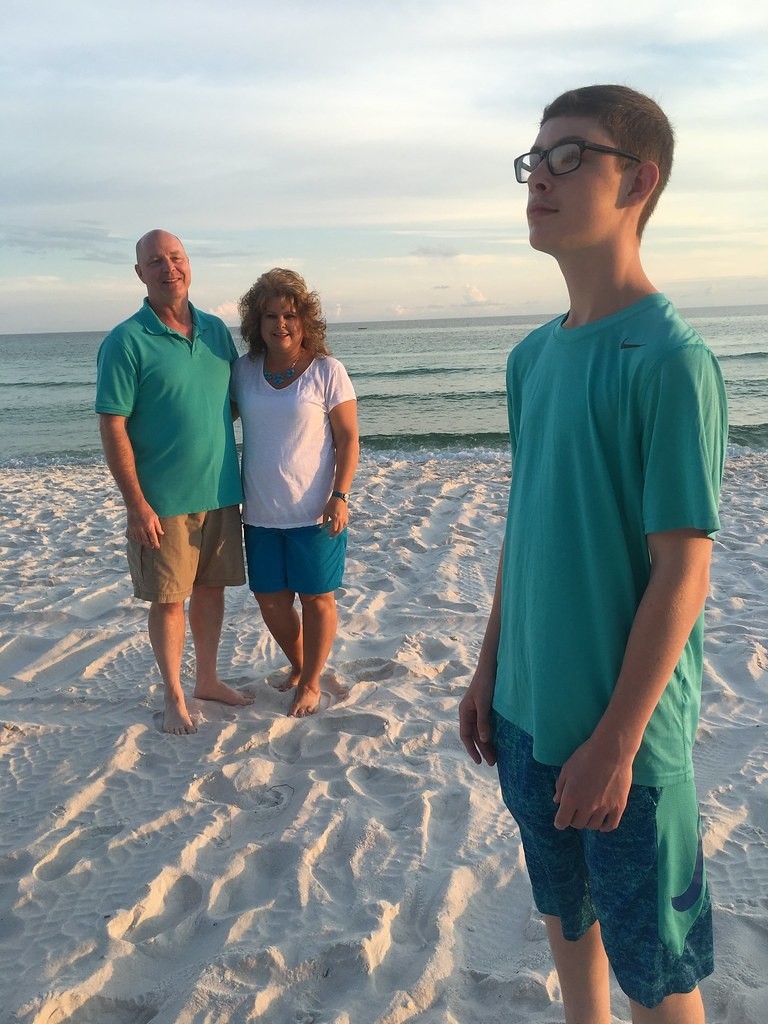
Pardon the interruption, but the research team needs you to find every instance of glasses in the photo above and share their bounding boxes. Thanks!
[514,140,642,184]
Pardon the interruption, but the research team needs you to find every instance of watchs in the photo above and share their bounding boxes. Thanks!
[332,491,350,502]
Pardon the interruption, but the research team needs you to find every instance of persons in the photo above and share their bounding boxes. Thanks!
[230,268,358,716]
[459,84,731,1024]
[94,229,253,737]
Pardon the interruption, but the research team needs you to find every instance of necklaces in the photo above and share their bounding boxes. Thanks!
[264,357,300,384]
